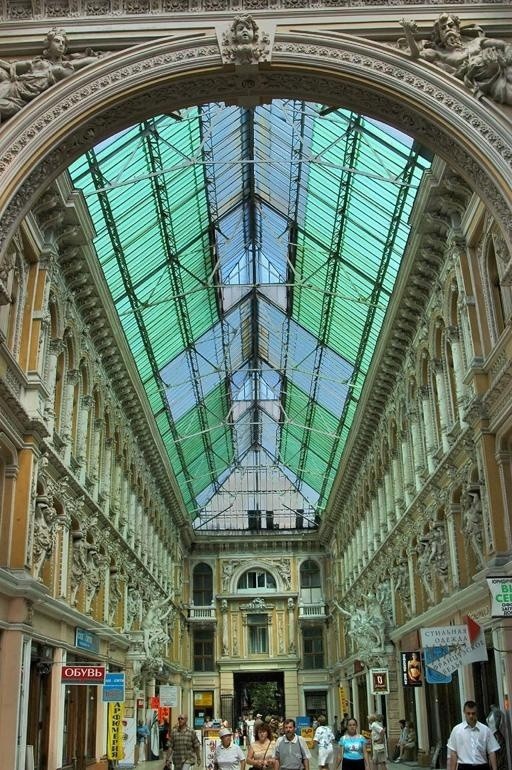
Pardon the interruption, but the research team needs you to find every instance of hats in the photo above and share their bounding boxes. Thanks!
[218,728,233,738]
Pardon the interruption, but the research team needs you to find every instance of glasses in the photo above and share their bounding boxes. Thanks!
[178,717,187,720]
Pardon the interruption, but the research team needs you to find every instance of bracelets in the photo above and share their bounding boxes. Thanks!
[166,760,170,761]
[197,759,200,760]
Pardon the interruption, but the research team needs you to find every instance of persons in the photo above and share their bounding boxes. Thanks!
[137,720,150,762]
[160,716,171,751]
[332,489,486,653]
[227,14,260,42]
[486,702,508,770]
[408,652,422,683]
[399,12,511,106]
[201,712,417,770]
[165,714,201,770]
[446,700,501,770]
[0,27,100,123]
[33,502,176,660]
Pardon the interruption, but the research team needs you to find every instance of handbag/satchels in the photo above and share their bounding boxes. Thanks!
[249,768,263,770]
